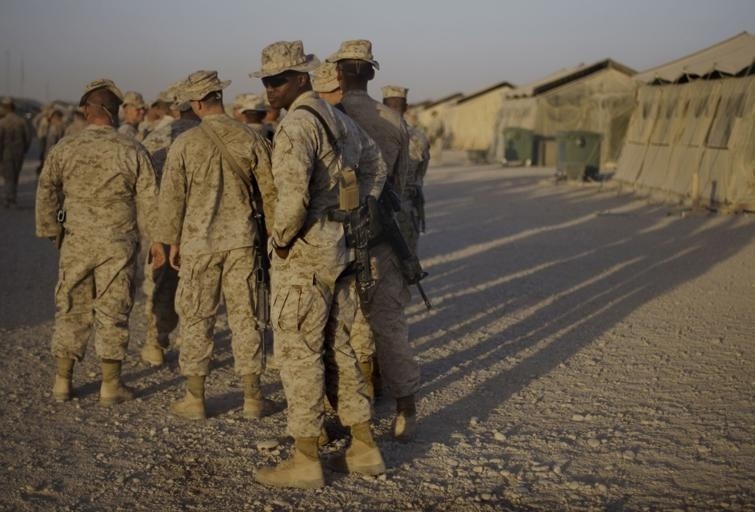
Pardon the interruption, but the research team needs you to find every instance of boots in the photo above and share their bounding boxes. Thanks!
[141,323,165,368]
[99,358,143,408]
[169,375,208,422]
[52,357,76,403]
[243,375,416,489]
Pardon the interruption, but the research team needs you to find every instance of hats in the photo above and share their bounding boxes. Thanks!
[151,71,232,112]
[381,85,410,99]
[232,40,379,114]
[48,78,148,118]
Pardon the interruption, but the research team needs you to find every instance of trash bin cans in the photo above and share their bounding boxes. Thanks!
[553,129,604,181]
[500,126,535,168]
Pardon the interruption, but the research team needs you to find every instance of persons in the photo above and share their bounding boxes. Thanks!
[1,95,32,207]
[32,70,282,419]
[245,40,433,489]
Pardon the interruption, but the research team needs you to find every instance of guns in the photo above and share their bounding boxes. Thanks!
[328,195,379,306]
[378,190,432,311]
[248,171,271,371]
